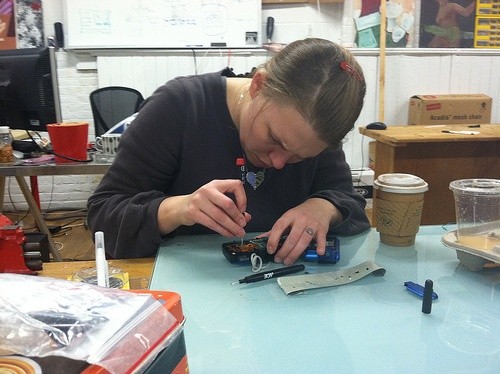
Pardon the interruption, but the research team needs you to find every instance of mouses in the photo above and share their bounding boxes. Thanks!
[366,122,386,130]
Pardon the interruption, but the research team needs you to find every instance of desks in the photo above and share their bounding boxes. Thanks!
[146,234,500,374]
[0,152,114,259]
[33,251,160,301]
[360,125,500,223]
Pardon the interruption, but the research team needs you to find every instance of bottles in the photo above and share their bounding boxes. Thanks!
[0,126,13,163]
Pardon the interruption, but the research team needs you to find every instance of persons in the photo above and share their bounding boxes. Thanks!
[428,0,475,48]
[87,38,372,266]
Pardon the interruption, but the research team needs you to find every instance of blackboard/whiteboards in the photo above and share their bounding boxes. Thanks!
[61,0,263,49]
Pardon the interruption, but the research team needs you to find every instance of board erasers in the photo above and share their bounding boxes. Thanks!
[211,43,226,46]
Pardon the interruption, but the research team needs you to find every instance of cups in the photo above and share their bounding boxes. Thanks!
[449,179,500,250]
[373,173,429,246]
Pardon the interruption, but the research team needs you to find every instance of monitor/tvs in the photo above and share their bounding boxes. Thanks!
[0,47,63,132]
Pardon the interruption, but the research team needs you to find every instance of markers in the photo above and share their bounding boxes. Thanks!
[239,264,305,284]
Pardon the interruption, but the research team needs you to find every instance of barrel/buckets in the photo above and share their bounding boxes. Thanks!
[47,123,89,162]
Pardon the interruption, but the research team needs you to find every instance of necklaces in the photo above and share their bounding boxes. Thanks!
[236,89,244,124]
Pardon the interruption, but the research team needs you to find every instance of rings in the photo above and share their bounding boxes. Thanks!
[306,228,313,235]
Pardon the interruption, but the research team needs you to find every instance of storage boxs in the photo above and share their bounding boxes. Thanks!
[0,289,190,374]
[351,167,375,210]
[409,94,492,125]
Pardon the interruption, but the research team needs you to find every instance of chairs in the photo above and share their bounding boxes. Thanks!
[90,87,145,138]
